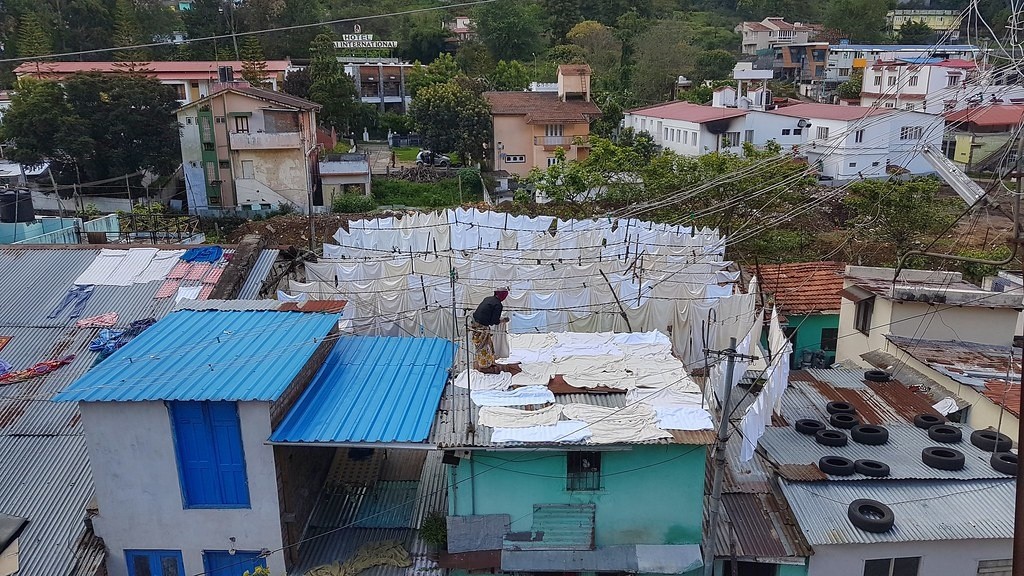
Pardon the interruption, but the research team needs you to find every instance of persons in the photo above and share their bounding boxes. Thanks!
[392,151,395,168]
[425,151,436,168]
[472,290,509,373]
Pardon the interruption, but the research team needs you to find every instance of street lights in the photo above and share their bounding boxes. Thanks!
[532,53,537,81]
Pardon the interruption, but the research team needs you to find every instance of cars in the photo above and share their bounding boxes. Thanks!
[414,150,452,167]
